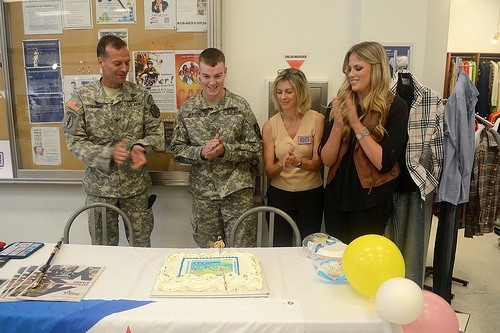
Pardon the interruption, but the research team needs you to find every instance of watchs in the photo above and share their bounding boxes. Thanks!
[295,160,302,167]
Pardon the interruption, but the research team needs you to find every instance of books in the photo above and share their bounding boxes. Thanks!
[0,264,107,303]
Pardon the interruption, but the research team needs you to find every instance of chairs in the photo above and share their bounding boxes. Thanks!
[230,205,301,247]
[61,202,134,246]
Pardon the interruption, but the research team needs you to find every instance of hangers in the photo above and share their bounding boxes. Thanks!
[397,67,412,91]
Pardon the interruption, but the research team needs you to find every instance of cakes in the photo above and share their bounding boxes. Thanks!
[154,251,263,292]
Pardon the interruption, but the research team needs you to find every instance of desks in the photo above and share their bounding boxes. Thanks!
[0,243,405,332]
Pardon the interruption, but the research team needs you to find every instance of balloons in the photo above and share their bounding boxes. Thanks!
[342,233,406,299]
[402,290,460,333]
[376,278,424,325]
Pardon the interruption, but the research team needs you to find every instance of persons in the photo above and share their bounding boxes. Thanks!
[179,62,200,85]
[167,48,264,247]
[151,0,169,13]
[71,81,77,89]
[139,61,161,90]
[319,40,409,247]
[262,68,328,247]
[63,35,165,247]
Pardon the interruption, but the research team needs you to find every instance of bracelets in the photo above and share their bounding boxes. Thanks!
[355,127,371,140]
